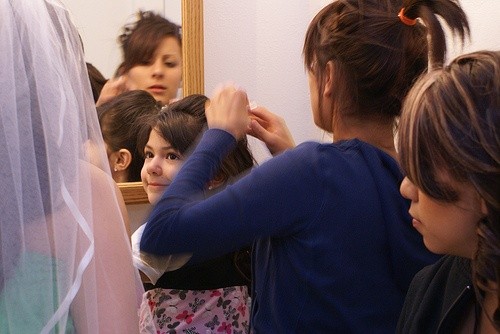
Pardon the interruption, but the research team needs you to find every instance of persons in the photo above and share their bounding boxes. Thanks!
[126,93,257,333]
[141,0,472,329]
[95,10,184,106]
[398,49,500,333]
[86,62,108,100]
[83,90,164,183]
[1,0,142,334]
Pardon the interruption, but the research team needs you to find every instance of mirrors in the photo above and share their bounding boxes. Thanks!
[62,0,205,203]
